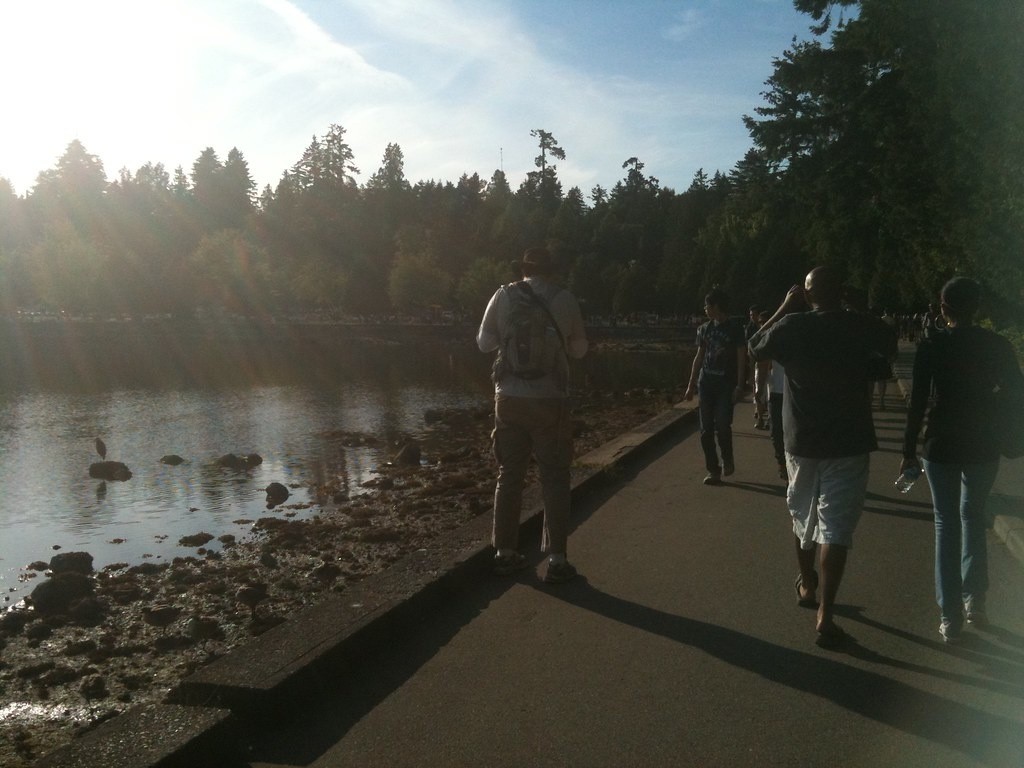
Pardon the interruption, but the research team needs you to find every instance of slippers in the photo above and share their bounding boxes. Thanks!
[815,622,845,646]
[794,571,818,606]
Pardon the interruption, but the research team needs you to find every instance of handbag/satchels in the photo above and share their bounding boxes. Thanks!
[992,334,1024,459]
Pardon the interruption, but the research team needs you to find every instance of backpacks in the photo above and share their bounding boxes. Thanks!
[491,283,562,381]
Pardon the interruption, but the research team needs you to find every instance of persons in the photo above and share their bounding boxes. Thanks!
[476,247,588,584]
[748,266,900,646]
[899,278,1021,644]
[845,303,939,410]
[685,289,813,485]
[585,311,703,328]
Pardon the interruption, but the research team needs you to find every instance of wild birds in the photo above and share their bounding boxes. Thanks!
[92,437,106,461]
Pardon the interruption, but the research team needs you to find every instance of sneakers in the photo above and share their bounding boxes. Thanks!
[494,551,528,576]
[545,560,577,583]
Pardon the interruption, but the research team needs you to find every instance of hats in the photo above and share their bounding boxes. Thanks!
[512,247,560,278]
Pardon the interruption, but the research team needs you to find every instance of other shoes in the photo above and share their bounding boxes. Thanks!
[778,464,787,479]
[967,609,986,624]
[723,456,734,476]
[939,622,961,643]
[755,420,770,429]
[703,473,721,484]
